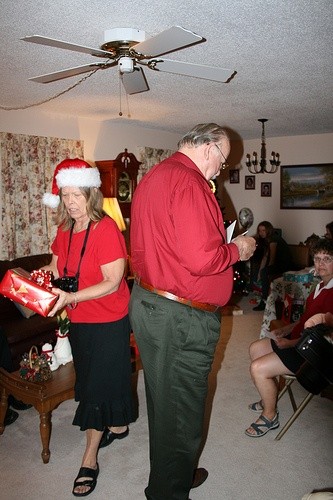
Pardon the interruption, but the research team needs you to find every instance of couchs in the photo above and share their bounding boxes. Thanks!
[0,253,53,365]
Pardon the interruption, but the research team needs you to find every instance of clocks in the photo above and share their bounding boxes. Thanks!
[95,147,144,218]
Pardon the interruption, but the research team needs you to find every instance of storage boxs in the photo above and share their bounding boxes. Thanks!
[0,266,59,317]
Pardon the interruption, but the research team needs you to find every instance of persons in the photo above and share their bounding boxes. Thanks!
[39,158,134,497]
[245,239,333,437]
[253,221,290,311]
[129,123,256,500]
[326,222,333,242]
[231,172,238,182]
[247,178,254,188]
[263,185,270,195]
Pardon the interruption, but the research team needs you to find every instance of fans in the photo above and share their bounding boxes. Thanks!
[19,26,235,117]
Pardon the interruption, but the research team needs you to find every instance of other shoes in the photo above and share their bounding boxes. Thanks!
[8,394,33,410]
[190,468,208,489]
[2,409,20,425]
[253,300,266,311]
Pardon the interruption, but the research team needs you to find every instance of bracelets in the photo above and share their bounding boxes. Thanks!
[68,292,77,310]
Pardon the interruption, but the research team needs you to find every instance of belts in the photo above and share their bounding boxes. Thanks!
[134,277,222,313]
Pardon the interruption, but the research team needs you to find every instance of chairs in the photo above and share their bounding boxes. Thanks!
[275,373,327,440]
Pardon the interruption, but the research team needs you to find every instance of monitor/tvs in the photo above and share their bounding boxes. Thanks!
[279,163,333,209]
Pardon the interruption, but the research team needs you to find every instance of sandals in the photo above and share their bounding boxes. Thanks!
[249,399,279,414]
[245,414,280,438]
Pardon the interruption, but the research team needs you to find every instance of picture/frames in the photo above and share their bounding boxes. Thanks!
[245,176,255,190]
[229,170,239,183]
[261,182,272,197]
[280,163,333,210]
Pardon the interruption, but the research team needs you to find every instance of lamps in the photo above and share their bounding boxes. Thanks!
[117,42,135,74]
[245,119,280,174]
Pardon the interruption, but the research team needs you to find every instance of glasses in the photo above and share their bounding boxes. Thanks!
[207,141,231,170]
[313,256,333,264]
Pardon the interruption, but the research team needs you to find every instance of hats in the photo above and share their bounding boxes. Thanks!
[41,157,102,209]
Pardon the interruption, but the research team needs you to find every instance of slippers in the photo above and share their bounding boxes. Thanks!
[73,461,99,497]
[99,426,129,447]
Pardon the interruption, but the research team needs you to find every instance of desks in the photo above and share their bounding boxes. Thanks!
[0,353,146,464]
